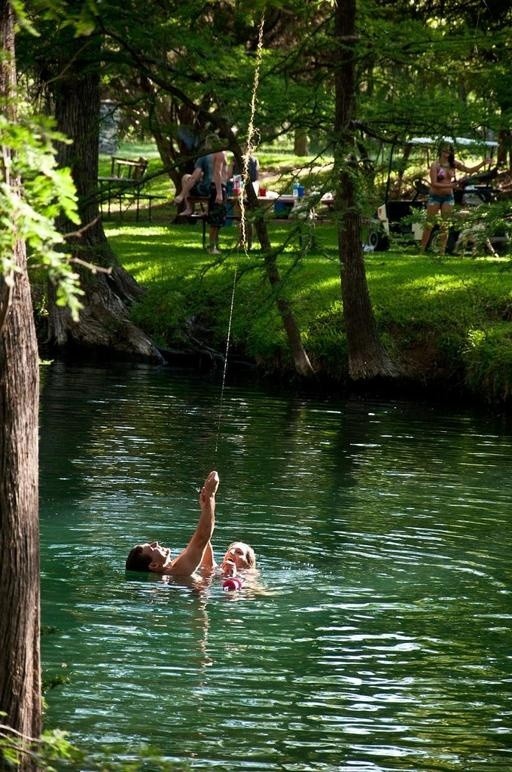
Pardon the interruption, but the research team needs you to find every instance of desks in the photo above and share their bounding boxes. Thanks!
[188,195,335,247]
[99,177,140,222]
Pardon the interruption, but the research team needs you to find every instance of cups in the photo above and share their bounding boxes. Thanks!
[293,185,304,197]
[260,187,266,196]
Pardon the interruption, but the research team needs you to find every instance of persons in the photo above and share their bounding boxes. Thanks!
[421,140,494,256]
[125,469,220,581]
[178,133,261,256]
[195,487,270,598]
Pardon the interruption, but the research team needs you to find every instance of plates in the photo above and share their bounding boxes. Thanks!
[281,195,293,198]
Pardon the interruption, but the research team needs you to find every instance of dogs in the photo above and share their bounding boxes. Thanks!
[453,210,499,258]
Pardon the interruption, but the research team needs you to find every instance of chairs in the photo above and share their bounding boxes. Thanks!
[108,157,148,217]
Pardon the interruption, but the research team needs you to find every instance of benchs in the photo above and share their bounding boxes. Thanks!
[192,214,331,251]
[116,193,167,223]
